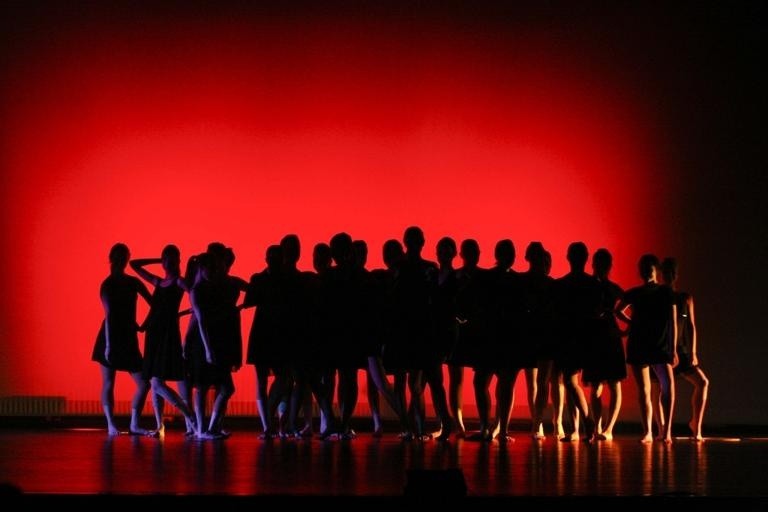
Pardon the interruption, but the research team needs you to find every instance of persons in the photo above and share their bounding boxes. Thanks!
[91,243,154,436]
[653,256,709,441]
[172,228,634,445]
[129,244,193,437]
[614,254,681,445]
